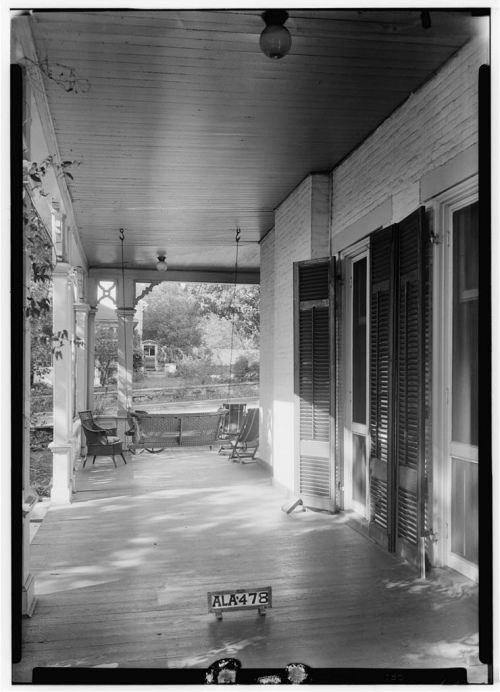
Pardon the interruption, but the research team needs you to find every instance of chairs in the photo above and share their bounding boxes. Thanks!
[217,403,259,465]
[77,410,127,468]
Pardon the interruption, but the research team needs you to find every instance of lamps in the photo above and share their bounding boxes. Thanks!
[259,11,292,59]
[156,256,168,272]
